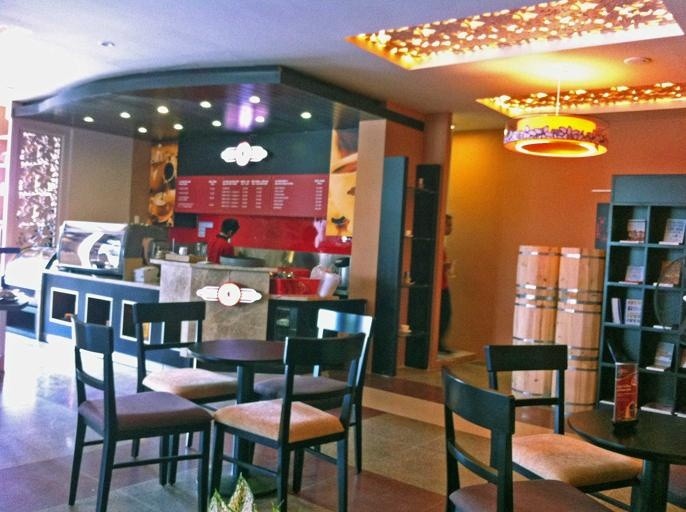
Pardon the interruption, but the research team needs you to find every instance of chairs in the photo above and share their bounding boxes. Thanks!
[68,313,214,512]
[215,331,366,512]
[441,345,645,512]
[131,300,239,486]
[246,309,376,473]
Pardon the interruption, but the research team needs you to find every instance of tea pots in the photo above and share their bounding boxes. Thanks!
[163,155,176,189]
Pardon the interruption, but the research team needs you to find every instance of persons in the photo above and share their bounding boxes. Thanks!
[439,215,454,355]
[208,218,240,263]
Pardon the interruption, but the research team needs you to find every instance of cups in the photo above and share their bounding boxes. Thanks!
[149,192,171,216]
[179,247,188,256]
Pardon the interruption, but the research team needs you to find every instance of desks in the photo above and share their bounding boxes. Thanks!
[566,406,686,511]
[185,339,286,499]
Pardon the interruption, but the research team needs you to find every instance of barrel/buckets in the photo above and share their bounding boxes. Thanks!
[510,245,603,413]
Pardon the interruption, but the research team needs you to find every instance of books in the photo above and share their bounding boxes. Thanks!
[610,218,686,373]
[640,402,674,415]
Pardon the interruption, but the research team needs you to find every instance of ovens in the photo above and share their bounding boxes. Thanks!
[56,220,167,281]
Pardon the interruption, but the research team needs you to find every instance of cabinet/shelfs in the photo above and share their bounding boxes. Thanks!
[593,174,686,408]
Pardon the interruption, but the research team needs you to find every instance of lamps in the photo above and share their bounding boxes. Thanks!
[503,76,612,160]
